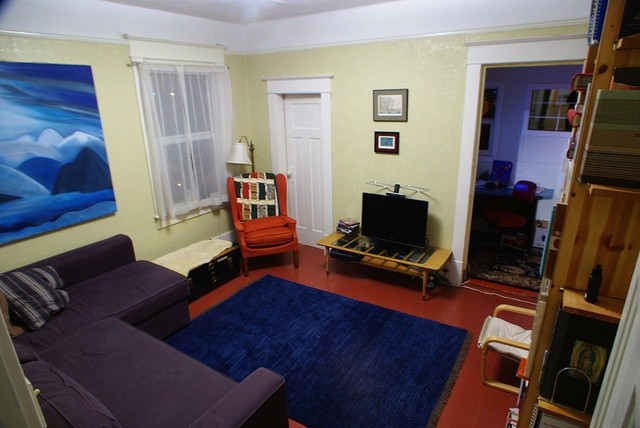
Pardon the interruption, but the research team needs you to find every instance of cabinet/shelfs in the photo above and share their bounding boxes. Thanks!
[506,0,640,428]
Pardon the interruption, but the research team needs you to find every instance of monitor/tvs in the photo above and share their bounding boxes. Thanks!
[361,191,429,256]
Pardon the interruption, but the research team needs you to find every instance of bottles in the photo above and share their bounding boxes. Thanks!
[584,262,603,303]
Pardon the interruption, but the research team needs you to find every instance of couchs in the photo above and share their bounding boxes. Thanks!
[227,173,299,277]
[0,234,290,428]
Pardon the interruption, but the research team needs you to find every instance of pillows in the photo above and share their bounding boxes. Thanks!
[0,265,70,330]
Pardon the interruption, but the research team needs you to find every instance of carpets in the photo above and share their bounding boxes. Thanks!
[471,247,543,293]
[161,274,473,427]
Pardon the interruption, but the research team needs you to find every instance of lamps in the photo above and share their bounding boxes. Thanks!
[225,136,254,174]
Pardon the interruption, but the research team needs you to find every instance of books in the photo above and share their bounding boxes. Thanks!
[336,217,360,236]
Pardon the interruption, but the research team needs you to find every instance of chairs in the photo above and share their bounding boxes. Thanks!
[487,181,536,269]
[477,305,537,396]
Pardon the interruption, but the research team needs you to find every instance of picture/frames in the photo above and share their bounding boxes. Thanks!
[374,132,400,154]
[372,88,408,122]
[477,80,506,162]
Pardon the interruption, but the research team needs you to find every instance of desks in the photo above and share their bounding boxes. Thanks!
[477,184,554,255]
